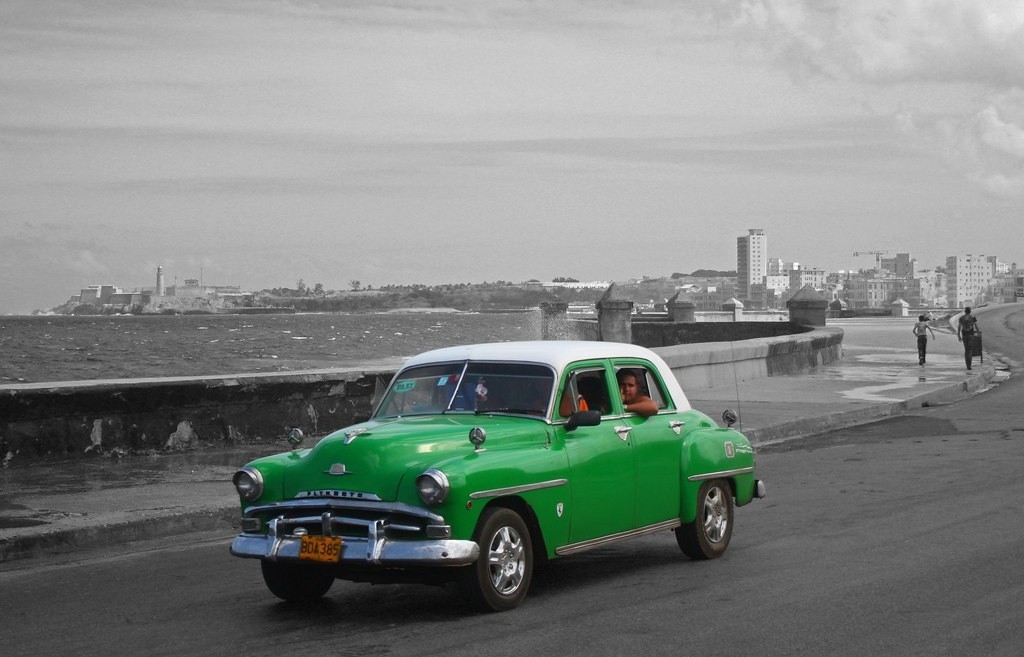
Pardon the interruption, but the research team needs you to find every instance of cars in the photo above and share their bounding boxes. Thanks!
[230,341,766,614]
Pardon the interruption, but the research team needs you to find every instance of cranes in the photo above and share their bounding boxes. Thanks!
[853,250,963,269]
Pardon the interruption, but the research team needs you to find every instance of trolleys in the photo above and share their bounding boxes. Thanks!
[960,331,983,364]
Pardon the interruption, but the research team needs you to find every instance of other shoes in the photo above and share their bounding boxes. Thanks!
[919,358,925,365]
[967,365,971,370]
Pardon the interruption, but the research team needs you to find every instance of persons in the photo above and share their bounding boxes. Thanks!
[913,315,935,365]
[958,306,980,369]
[589,370,658,416]
[924,310,932,322]
[530,377,589,416]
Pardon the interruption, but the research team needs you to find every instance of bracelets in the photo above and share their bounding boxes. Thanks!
[623,403,627,409]
